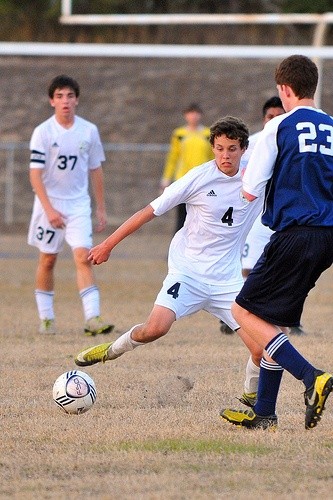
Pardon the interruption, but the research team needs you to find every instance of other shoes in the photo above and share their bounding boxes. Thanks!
[219,319,233,334]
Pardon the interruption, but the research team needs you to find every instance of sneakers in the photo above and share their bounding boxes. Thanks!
[85,317,114,335]
[220,407,277,433]
[40,319,56,335]
[304,370,333,430]
[74,341,114,366]
[238,393,257,406]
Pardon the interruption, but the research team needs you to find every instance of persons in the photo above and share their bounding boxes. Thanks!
[220,96,304,336]
[221,55,333,432]
[73,117,267,406]
[160,104,215,232]
[27,74,114,336]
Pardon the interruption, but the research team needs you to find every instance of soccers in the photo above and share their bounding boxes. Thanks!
[52,370,96,415]
